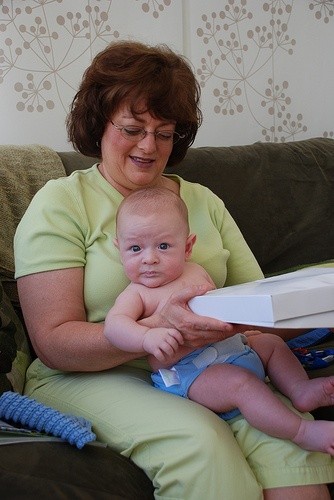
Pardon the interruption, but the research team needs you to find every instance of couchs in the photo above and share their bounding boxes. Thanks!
[0,137,334,500]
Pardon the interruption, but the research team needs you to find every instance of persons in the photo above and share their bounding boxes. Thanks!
[14,41,334,500]
[105,188,334,456]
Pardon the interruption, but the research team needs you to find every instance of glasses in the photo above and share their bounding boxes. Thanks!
[109,119,181,145]
